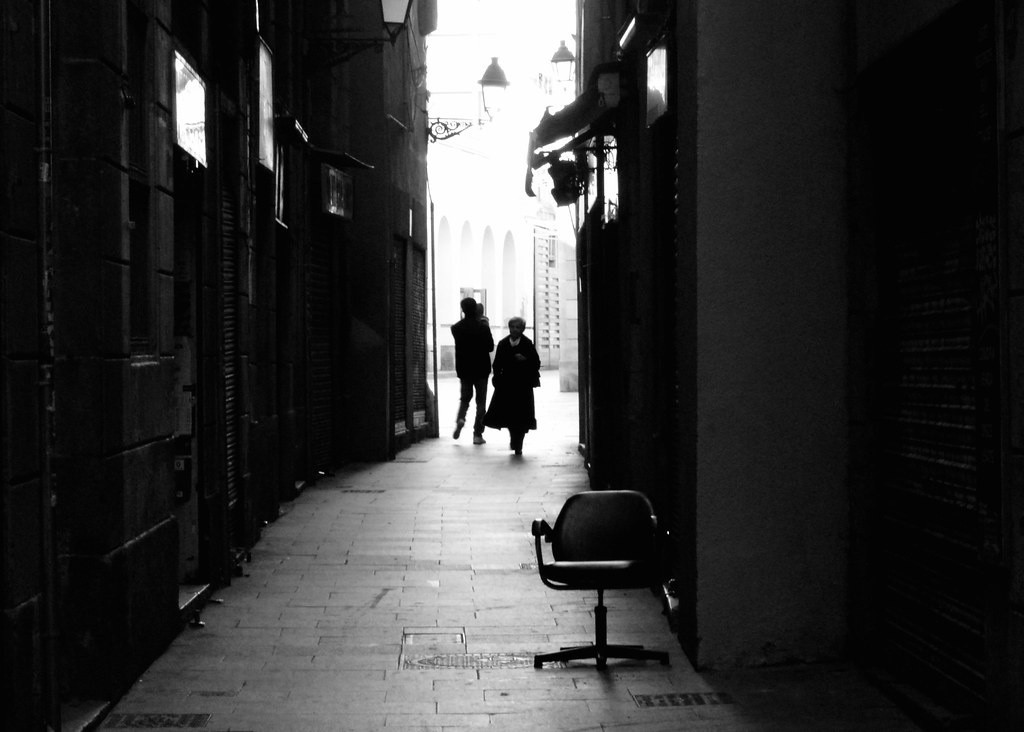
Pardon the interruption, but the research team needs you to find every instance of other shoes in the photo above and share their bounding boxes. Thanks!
[515,450,522,454]
[510,443,515,450]
[453,420,465,439]
[473,436,486,444]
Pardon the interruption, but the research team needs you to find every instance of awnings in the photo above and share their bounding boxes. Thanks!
[524,85,605,197]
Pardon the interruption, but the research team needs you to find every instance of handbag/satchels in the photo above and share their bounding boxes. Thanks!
[526,361,540,391]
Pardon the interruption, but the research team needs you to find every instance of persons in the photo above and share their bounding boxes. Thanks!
[450,296,496,444]
[480,316,543,456]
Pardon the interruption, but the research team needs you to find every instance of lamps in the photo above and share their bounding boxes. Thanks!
[550,39,577,92]
[428,57,510,144]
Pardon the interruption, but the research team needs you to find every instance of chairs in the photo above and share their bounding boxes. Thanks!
[532,490,672,674]
